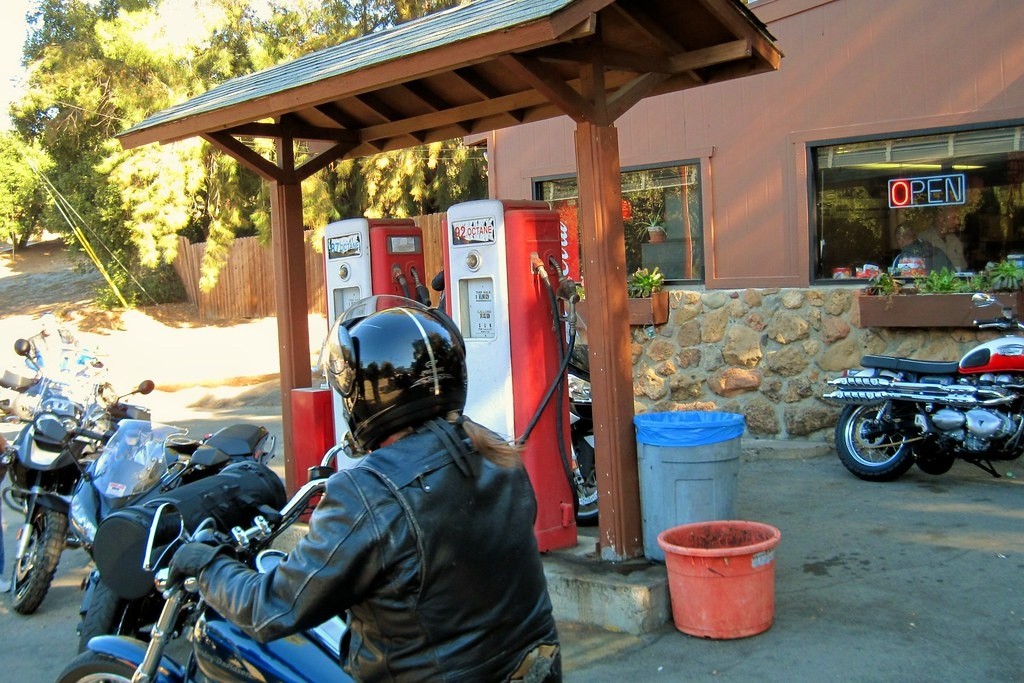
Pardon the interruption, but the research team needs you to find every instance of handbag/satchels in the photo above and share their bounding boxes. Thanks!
[93,460,288,600]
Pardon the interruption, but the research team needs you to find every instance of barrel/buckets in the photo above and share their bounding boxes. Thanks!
[658,521,781,640]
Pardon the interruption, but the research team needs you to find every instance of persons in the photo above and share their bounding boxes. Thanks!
[165,294,565,683]
[891,207,968,277]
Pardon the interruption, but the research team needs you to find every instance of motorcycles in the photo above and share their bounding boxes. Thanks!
[55,419,357,683]
[0,327,152,617]
[822,292,1024,483]
[564,313,600,530]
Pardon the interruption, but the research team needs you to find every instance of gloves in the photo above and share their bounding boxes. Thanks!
[166,543,239,591]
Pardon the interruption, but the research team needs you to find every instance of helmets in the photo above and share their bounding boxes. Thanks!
[329,308,468,448]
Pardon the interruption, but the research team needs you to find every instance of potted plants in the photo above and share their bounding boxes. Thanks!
[857,259,1024,327]
[633,205,671,244]
[565,266,670,325]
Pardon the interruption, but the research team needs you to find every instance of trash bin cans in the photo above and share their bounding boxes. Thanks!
[633,410,745,563]
[657,520,780,639]
